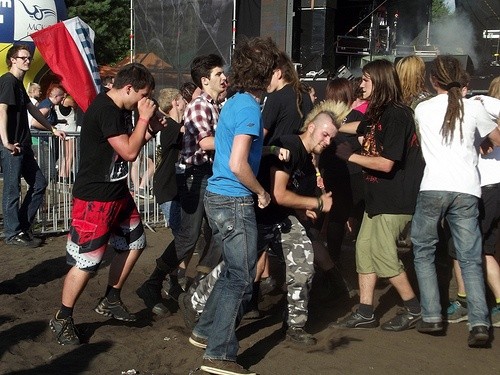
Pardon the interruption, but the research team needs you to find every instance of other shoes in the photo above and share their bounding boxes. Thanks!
[152,294,178,318]
[190,272,206,294]
[320,269,347,294]
[242,310,260,318]
[166,275,188,291]
[259,294,283,309]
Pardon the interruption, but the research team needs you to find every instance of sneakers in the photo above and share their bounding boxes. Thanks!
[164,282,184,300]
[416,319,443,332]
[23,231,41,247]
[441,301,469,323]
[200,358,256,375]
[380,306,422,331]
[179,292,201,328]
[5,232,31,245]
[337,311,378,329]
[487,305,500,327]
[48,309,79,345]
[95,296,136,322]
[469,325,490,348]
[286,328,316,345]
[188,335,208,349]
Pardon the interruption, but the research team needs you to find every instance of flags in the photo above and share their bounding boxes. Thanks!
[30,16,102,112]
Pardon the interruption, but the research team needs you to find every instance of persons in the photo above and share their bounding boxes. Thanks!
[0,45,66,248]
[136,51,500,347]
[49,63,167,346]
[189,37,290,375]
[27,82,77,184]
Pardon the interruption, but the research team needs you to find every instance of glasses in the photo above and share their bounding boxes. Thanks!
[13,56,34,64]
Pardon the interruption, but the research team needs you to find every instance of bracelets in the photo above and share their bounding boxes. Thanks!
[270,145,276,154]
[139,118,149,124]
[318,197,323,212]
